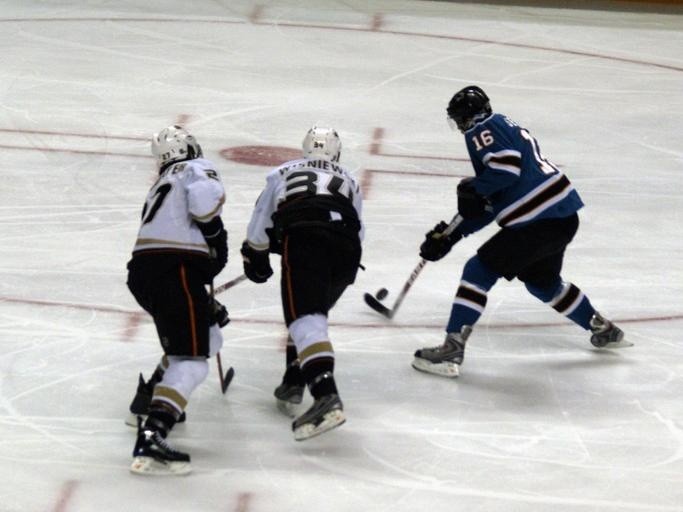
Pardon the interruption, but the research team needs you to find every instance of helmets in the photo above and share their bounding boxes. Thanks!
[445,85,491,133]
[302,125,340,164]
[151,127,201,173]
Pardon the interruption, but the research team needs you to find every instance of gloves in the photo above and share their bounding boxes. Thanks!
[203,225,226,265]
[456,178,485,220]
[206,298,230,328]
[418,221,460,261]
[239,239,272,283]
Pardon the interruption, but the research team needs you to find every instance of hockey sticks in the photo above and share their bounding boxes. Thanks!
[210,280,233,397]
[364,213,464,319]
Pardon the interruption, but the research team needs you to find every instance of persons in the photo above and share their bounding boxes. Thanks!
[414,85,623,365]
[240,127,364,431]
[125,126,232,462]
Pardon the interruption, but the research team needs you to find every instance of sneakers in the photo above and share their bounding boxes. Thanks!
[412,331,467,366]
[130,385,186,422]
[131,429,189,461]
[291,393,343,430]
[591,312,624,346]
[274,373,304,398]
